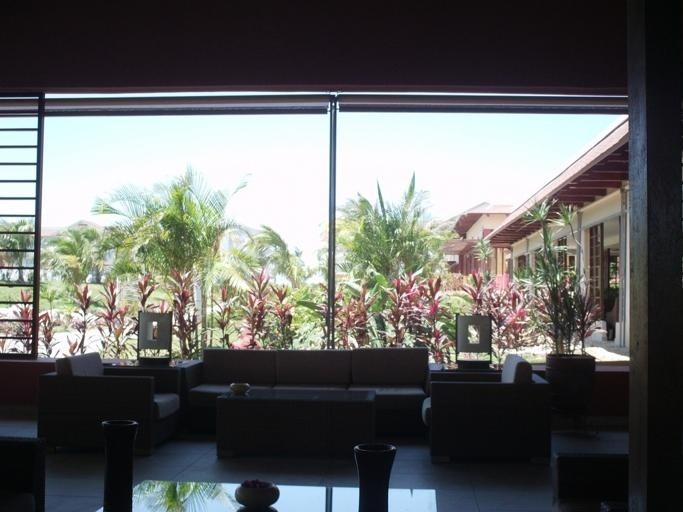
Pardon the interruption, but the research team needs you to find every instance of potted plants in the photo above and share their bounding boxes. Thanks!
[527,192,621,424]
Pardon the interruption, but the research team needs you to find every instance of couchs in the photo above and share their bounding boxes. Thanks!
[175,339,432,442]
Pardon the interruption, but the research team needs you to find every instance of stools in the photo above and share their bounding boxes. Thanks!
[547,440,630,504]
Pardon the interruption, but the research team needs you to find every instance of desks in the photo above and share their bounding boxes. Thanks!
[212,389,378,465]
[102,358,200,400]
[420,361,500,390]
[91,477,438,512]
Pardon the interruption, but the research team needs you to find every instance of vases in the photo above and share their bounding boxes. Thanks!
[353,439,397,512]
[98,416,141,512]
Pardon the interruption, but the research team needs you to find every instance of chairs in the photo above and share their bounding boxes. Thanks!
[31,350,181,459]
[0,430,51,512]
[417,356,558,471]
